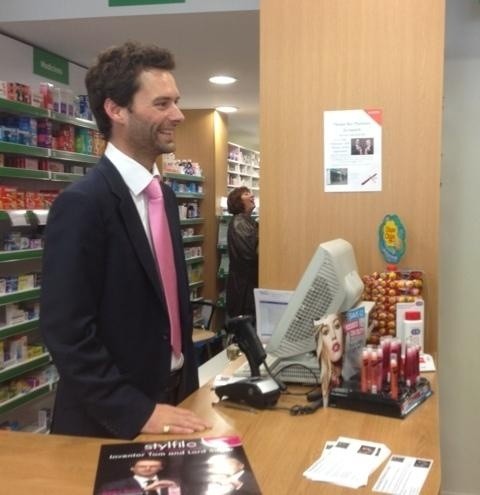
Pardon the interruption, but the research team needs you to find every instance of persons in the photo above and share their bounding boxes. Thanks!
[315,313,345,408]
[38,40,213,440]
[225,186,260,317]
[205,458,262,495]
[100,458,187,495]
[351,139,364,155]
[364,140,374,155]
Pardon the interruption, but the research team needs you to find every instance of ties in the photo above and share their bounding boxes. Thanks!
[147,480,158,495]
[145,177,184,360]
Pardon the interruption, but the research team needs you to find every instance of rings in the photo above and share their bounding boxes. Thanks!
[160,424,171,434]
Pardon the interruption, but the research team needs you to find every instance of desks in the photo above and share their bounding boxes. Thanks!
[1,344,442,495]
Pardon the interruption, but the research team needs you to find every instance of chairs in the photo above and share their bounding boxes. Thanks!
[186,301,216,369]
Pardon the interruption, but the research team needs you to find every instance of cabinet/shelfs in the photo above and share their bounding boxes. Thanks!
[217,141,261,356]
[0,87,213,435]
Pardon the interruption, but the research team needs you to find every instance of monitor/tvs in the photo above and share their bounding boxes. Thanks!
[233,237,377,385]
